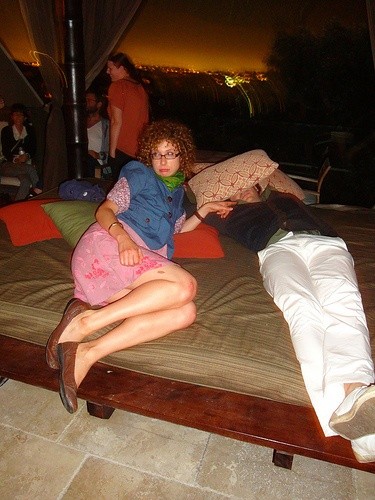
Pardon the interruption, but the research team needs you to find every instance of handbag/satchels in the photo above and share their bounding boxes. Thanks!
[58,178,106,201]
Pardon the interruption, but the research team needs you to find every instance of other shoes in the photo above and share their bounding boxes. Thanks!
[30,189,44,196]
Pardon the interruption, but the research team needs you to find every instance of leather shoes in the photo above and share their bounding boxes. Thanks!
[45,298,93,369]
[57,339,82,413]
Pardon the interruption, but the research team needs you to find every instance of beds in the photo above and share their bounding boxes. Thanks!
[1,177,375,476]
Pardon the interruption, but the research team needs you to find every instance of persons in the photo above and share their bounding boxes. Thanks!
[44,120,238,415]
[85,91,111,178]
[185,186,375,464]
[1,103,41,201]
[105,54,150,186]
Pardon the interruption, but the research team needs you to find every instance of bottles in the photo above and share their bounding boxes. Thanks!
[18,147,26,156]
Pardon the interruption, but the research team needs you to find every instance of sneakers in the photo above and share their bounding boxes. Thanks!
[328,385,375,463]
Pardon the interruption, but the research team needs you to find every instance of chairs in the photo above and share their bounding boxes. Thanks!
[286,162,332,204]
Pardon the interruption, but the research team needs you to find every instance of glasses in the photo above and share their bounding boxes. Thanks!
[150,152,181,160]
[87,98,96,102]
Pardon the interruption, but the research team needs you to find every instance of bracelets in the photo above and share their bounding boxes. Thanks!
[108,222,123,235]
[194,211,206,223]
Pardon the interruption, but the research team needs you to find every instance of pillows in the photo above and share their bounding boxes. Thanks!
[257,168,305,201]
[0,198,63,246]
[171,223,226,260]
[189,150,279,211]
[40,200,98,247]
